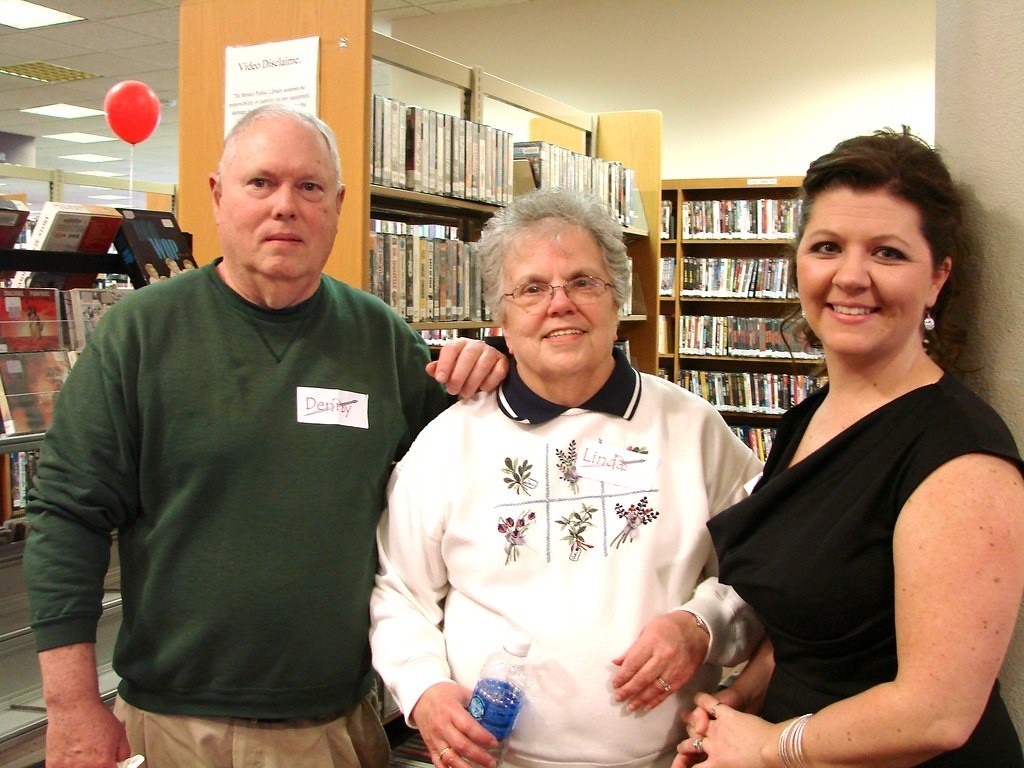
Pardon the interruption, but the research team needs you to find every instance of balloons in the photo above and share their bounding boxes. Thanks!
[104,81,162,145]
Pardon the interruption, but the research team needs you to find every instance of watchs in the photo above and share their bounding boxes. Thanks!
[695,615,709,633]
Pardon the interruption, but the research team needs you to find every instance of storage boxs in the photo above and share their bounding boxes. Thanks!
[0,198,200,436]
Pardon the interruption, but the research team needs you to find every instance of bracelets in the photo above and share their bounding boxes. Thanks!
[779,713,813,768]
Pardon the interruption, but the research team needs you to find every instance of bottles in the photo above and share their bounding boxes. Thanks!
[448,632,533,768]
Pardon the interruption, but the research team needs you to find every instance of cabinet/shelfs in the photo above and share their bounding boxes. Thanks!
[178,0,662,726]
[656,175,827,420]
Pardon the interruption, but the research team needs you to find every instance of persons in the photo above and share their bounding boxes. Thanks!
[24,102,512,768]
[165,258,183,277]
[183,259,195,269]
[370,186,767,767]
[674,124,1024,768]
[145,263,167,284]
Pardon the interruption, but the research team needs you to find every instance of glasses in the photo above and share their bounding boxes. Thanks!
[498,275,616,306]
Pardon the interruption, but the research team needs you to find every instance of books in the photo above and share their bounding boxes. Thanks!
[661,196,828,462]
[371,94,652,366]
[0,209,194,528]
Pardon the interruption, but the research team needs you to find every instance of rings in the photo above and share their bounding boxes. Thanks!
[438,747,450,758]
[659,677,671,691]
[693,736,704,752]
[711,703,721,714]
